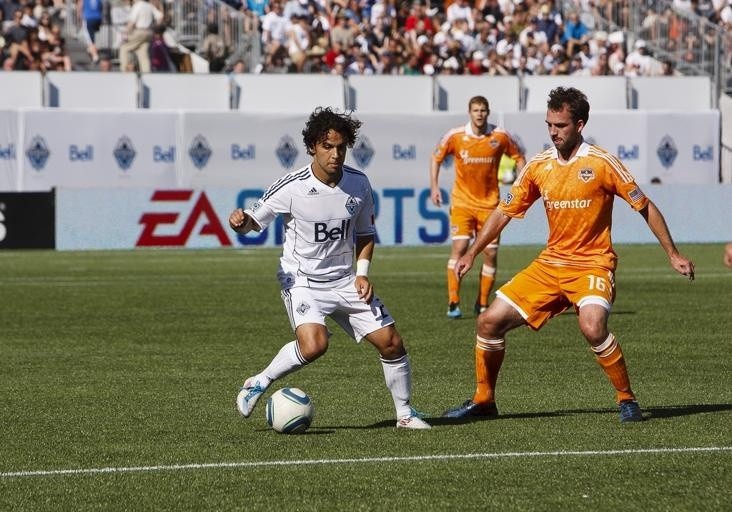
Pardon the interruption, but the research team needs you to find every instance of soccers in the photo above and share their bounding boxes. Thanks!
[266,387,314,433]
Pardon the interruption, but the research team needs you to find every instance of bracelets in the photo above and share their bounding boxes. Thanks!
[355,258,370,280]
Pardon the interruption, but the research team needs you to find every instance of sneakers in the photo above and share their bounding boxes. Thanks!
[392,411,433,432]
[233,373,268,419]
[474,301,489,316]
[445,301,464,319]
[617,401,645,424]
[438,397,501,426]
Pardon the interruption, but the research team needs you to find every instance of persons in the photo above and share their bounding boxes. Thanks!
[228,105,433,430]
[432,96,526,318]
[1,0,731,96]
[435,86,695,422]
[723,244,732,268]
